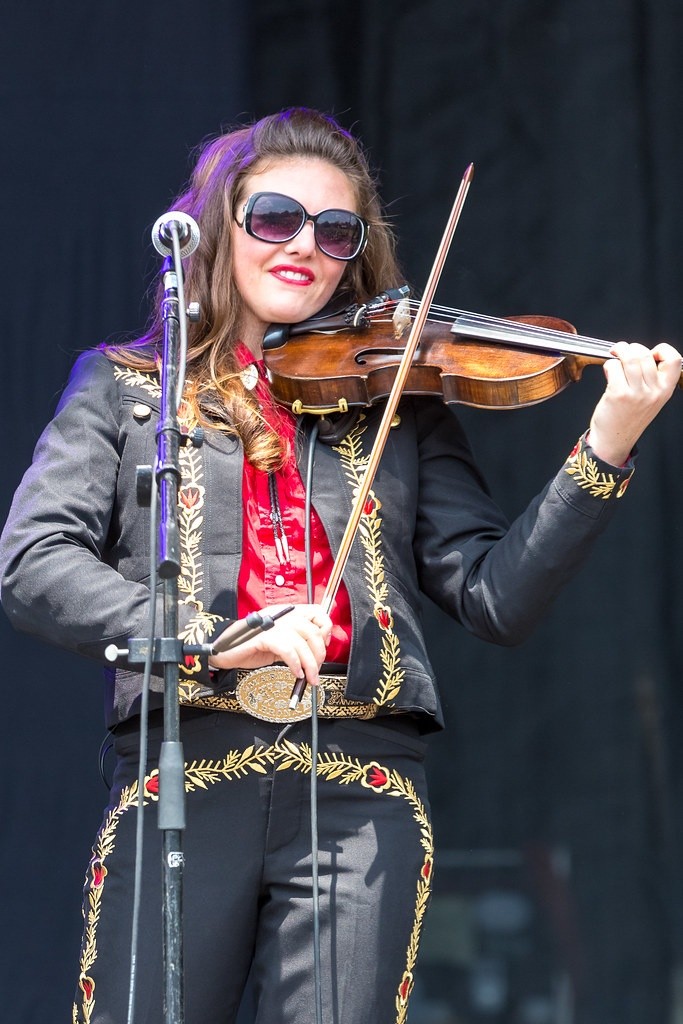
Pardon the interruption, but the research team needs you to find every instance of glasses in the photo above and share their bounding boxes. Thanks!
[234,192,369,261]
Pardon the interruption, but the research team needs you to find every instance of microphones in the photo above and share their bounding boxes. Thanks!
[152,211,200,259]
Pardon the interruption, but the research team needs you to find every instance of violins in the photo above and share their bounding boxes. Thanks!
[260,285,683,416]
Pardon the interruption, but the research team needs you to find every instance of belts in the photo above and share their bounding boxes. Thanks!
[177,667,409,724]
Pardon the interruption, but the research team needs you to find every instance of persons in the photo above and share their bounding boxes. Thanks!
[1,105,683,1024]
